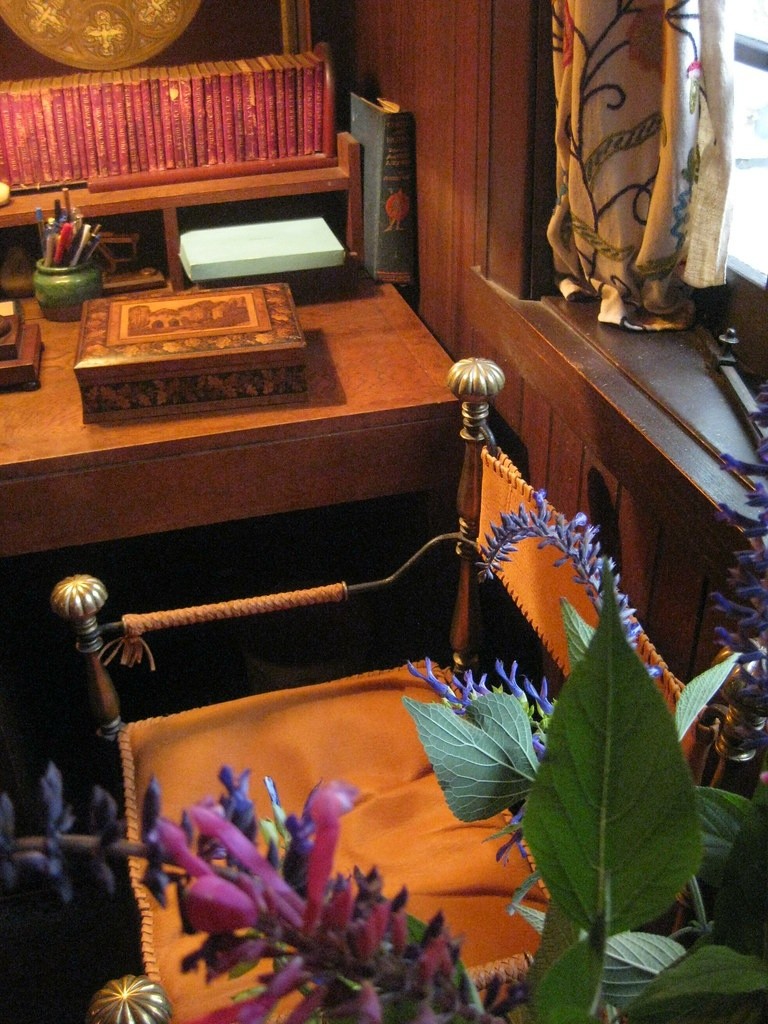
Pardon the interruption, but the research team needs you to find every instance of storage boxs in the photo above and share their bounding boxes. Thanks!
[74,283,309,422]
[178,212,350,306]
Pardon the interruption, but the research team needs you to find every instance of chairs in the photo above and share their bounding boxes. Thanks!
[52,356,768,1024]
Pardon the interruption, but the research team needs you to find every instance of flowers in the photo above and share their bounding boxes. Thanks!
[149,489,768,1024]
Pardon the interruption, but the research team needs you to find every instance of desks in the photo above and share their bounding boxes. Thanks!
[0,132,470,560]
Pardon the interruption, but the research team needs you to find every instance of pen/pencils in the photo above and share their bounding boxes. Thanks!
[34,187,103,267]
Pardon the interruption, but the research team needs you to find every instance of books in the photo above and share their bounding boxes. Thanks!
[0,50,324,190]
[350,90,416,284]
